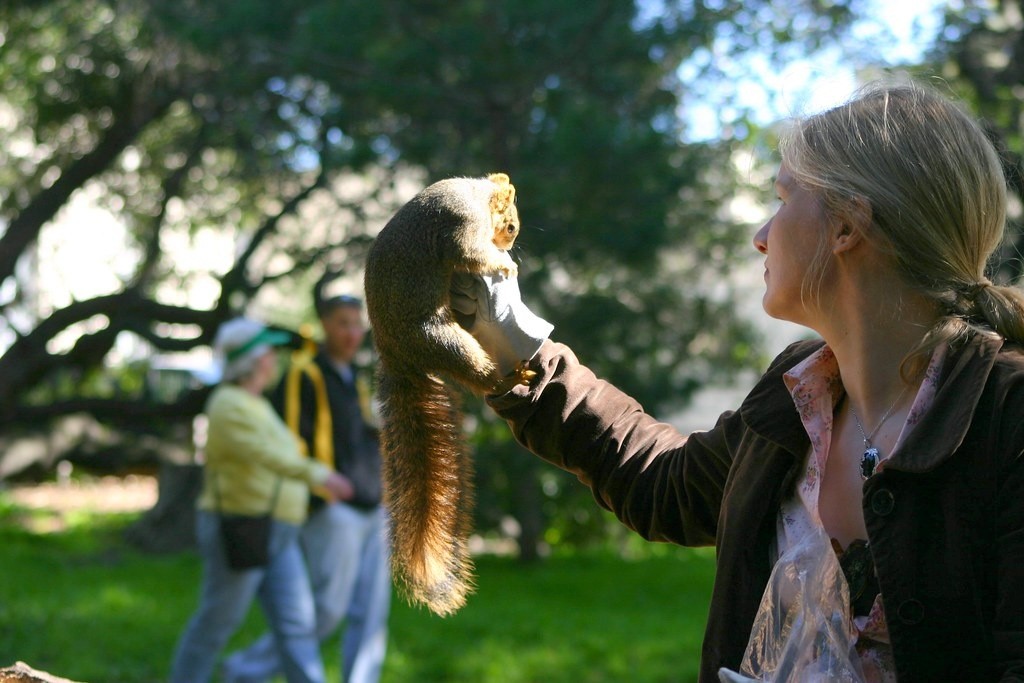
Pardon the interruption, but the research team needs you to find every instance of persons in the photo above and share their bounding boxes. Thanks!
[166,295,392,683]
[444,85,1024,683]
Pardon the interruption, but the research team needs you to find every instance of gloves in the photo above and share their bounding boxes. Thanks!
[449,254,557,377]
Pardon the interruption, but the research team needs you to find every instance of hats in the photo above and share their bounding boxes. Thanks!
[218,319,291,377]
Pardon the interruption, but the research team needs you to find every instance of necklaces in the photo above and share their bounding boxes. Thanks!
[848,352,936,481]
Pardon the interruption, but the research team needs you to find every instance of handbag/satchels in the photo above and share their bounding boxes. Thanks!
[218,511,275,572]
